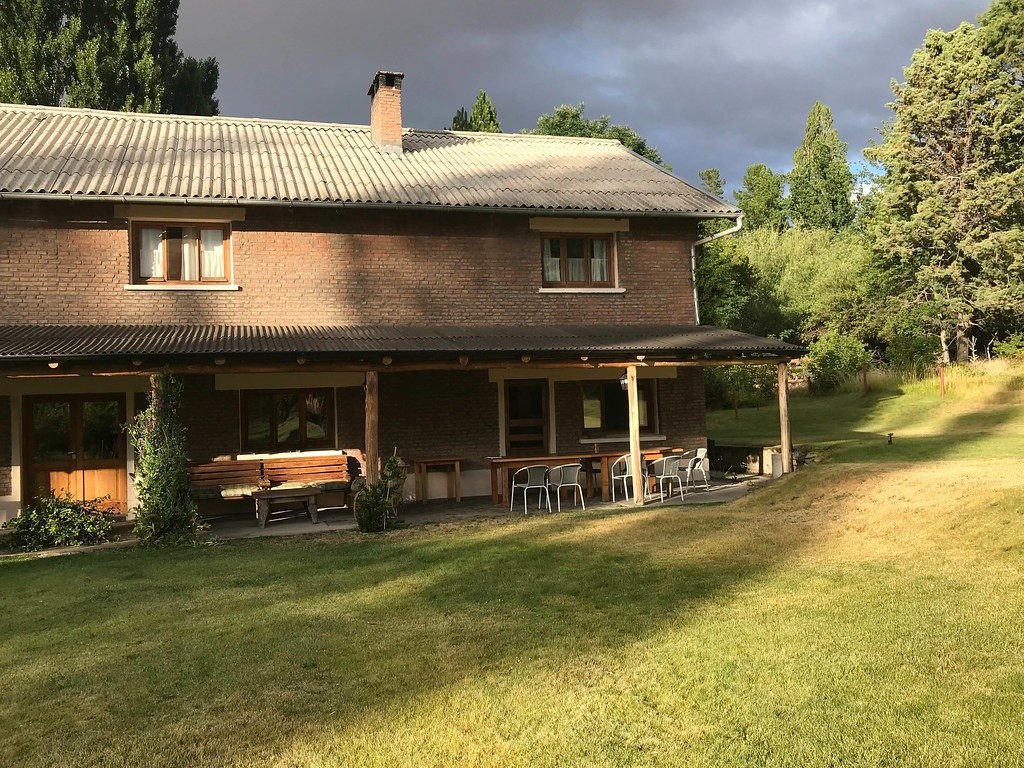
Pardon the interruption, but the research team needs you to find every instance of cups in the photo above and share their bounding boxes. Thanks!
[593,444,599,453]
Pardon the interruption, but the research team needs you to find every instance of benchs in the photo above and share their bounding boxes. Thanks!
[188,454,351,514]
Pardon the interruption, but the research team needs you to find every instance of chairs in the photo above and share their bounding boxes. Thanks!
[510,464,552,514]
[580,469,601,495]
[384,457,410,517]
[545,463,586,512]
[644,456,684,504]
[679,448,709,494]
[611,453,648,502]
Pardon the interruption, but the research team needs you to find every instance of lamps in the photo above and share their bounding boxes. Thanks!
[618,373,628,391]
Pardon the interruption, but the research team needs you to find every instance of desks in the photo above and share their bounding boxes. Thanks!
[411,456,465,505]
[251,487,323,528]
[484,447,685,508]
